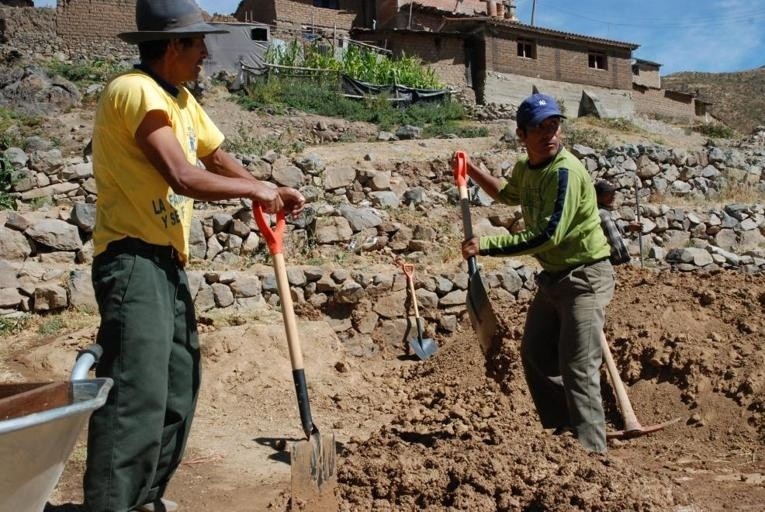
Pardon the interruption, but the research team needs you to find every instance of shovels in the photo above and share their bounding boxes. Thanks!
[402,152,497,361]
[251,200,337,512]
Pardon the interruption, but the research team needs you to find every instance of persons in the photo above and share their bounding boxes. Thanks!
[594,182,644,264]
[449,95,616,458]
[83,1,304,512]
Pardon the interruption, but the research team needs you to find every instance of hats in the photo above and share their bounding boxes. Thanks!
[517,94,567,128]
[117,0,230,45]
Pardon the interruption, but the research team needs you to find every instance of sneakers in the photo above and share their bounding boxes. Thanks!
[137,497,178,511]
